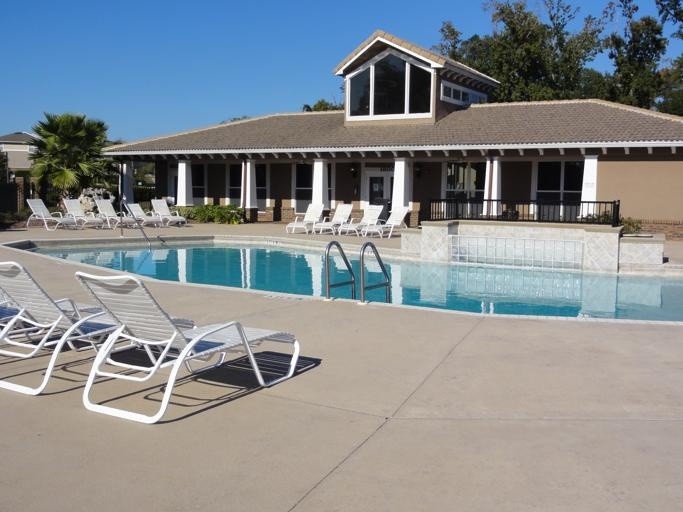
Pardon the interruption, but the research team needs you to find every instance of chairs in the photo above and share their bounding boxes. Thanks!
[25,198,187,230]
[0,263,196,398]
[284,203,411,240]
[75,270,302,425]
[0,289,105,336]
